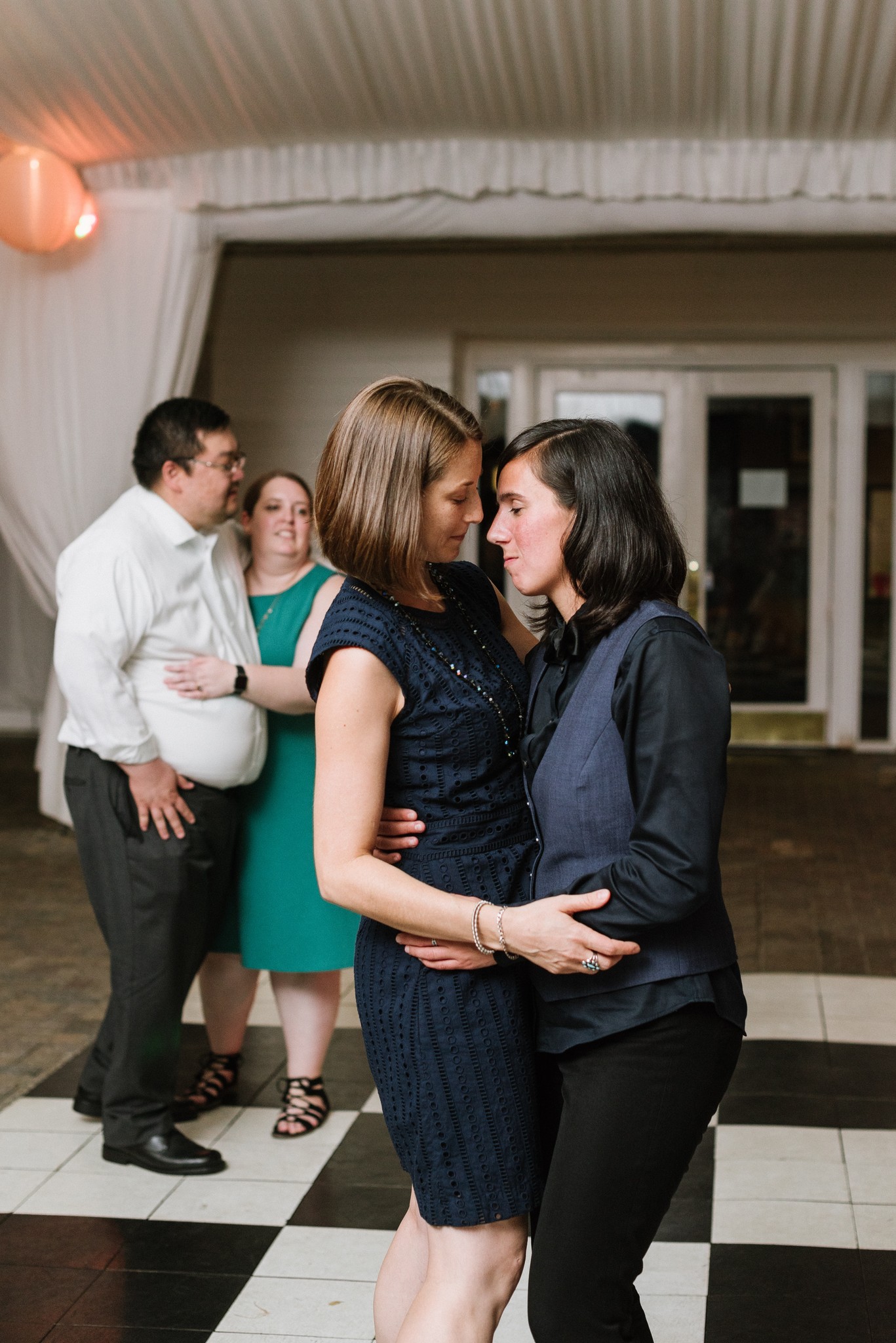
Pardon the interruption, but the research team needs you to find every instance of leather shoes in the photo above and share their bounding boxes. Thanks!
[102,1125,225,1175]
[71,1094,200,1122]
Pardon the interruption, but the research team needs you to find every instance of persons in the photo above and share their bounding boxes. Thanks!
[372,415,751,1340]
[165,468,370,1139]
[51,393,317,1179]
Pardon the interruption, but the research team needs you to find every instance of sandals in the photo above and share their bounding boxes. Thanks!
[180,1051,240,1111]
[271,1076,331,1140]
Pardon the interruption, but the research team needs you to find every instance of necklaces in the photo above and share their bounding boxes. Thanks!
[245,569,300,634]
[372,561,525,762]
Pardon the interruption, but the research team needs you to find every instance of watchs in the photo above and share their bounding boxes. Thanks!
[231,663,249,697]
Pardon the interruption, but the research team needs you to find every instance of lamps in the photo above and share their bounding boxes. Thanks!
[0,144,88,253]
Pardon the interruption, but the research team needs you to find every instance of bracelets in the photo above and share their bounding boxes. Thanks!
[471,900,519,961]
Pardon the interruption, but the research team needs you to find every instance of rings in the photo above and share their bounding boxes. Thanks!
[582,951,601,972]
[301,372,646,1340]
[431,938,437,947]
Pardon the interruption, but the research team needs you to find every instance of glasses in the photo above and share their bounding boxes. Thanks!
[175,451,247,475]
